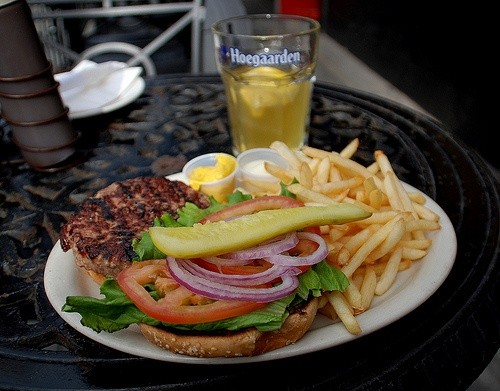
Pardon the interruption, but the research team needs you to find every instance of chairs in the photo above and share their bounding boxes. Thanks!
[29,0,208,76]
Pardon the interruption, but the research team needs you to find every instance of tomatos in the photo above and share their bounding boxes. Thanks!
[116,259,273,324]
[197,195,326,283]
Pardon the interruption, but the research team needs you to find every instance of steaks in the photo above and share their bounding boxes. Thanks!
[60,175,210,279]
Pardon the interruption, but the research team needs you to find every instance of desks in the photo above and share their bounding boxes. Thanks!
[0,76,500,391]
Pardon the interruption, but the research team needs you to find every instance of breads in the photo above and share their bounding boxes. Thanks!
[135,290,321,358]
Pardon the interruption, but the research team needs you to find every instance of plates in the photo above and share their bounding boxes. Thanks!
[53,67,145,118]
[43,171,457,364]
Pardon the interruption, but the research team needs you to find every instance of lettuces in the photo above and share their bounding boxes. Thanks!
[58,178,350,333]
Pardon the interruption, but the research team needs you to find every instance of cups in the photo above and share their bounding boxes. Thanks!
[184,153,237,204]
[211,14,320,156]
[237,149,292,196]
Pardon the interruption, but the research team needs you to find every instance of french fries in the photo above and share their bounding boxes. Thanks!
[264,137,442,335]
